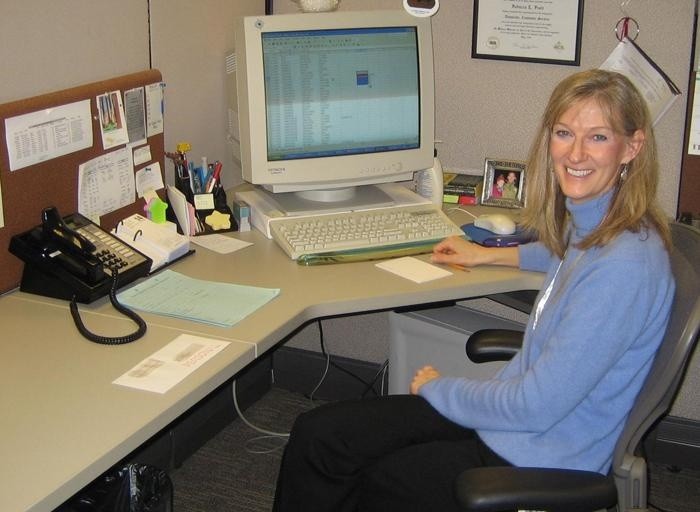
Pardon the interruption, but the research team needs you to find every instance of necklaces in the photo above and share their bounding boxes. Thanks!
[532,248,569,330]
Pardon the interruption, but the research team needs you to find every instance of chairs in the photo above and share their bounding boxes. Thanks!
[453,219,699,512]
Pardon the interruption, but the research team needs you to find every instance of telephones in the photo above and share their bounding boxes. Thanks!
[7,205,153,345]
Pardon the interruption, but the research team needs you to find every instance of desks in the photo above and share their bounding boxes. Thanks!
[1,172,547,511]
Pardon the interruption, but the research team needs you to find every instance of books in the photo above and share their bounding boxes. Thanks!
[166,183,203,236]
[116,269,280,327]
[110,214,196,276]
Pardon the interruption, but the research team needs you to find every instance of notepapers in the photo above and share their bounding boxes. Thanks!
[205,210,231,231]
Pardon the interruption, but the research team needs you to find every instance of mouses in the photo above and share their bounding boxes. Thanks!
[473,213,516,236]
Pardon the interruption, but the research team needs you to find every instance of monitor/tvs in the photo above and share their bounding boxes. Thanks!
[224,8,437,215]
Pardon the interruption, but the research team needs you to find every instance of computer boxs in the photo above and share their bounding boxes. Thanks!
[387,302,528,398]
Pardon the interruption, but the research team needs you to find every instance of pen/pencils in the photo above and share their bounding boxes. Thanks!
[188,156,223,196]
[447,264,471,272]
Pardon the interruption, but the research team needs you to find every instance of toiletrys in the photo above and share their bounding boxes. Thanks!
[414,147,445,212]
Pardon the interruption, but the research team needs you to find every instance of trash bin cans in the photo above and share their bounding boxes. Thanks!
[77,462,174,512]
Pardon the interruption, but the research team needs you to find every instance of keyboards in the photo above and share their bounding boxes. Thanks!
[269,204,465,261]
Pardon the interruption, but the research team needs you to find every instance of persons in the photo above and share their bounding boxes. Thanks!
[502,171,518,198]
[271,70,676,512]
[493,175,506,198]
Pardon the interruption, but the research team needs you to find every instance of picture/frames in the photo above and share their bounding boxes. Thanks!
[479,156,530,211]
[470,0,585,68]
